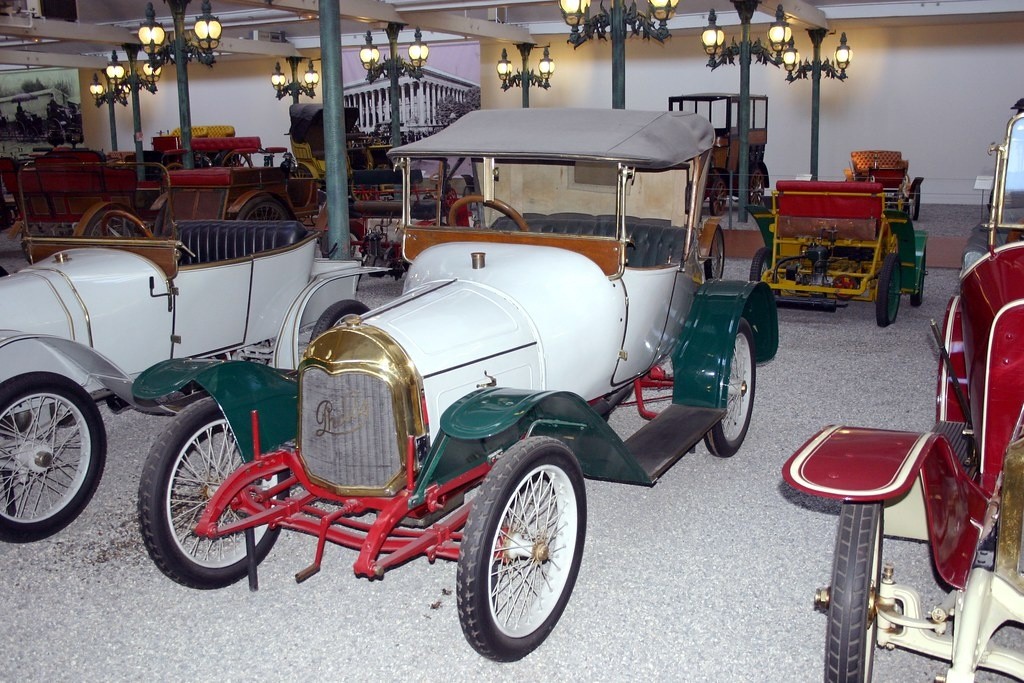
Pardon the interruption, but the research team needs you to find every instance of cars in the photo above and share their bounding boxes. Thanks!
[667,92,770,217]
[0,102,478,281]
[786,92,1024,683]
[0,160,365,544]
[747,178,930,328]
[843,151,926,222]
[135,104,782,665]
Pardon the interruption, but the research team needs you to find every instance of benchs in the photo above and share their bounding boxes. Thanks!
[350,168,441,213]
[850,149,908,176]
[149,218,309,266]
[776,178,888,239]
[492,216,690,269]
[0,124,326,198]
